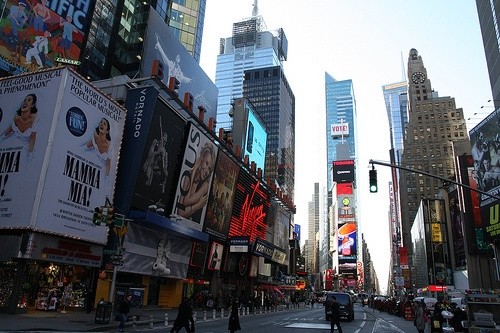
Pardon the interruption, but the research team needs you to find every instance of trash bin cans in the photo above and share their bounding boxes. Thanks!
[94,298,113,325]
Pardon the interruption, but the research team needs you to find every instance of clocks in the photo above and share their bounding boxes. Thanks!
[412,72,425,84]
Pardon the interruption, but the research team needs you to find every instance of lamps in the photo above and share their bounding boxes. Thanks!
[148,205,183,222]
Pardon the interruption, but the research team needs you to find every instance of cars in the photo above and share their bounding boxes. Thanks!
[354,293,389,313]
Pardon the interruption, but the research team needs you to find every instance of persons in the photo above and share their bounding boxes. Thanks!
[0,94,39,176]
[330,296,342,333]
[197,292,320,314]
[170,296,195,333]
[117,294,132,332]
[155,34,196,92]
[211,189,231,231]
[472,131,500,189]
[210,244,221,269]
[361,295,469,333]
[48,290,62,309]
[144,132,171,188]
[81,118,112,184]
[178,143,216,223]
[228,302,241,333]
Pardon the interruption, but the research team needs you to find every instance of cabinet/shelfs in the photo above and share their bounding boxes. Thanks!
[60,264,92,309]
[467,302,500,333]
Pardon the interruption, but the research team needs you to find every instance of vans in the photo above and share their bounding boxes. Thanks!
[323,292,356,322]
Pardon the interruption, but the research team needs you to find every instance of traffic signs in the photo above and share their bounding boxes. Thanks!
[112,260,124,266]
[109,255,123,260]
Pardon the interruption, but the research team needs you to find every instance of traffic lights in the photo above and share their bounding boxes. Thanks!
[369,170,378,193]
[92,206,103,226]
[106,205,116,226]
